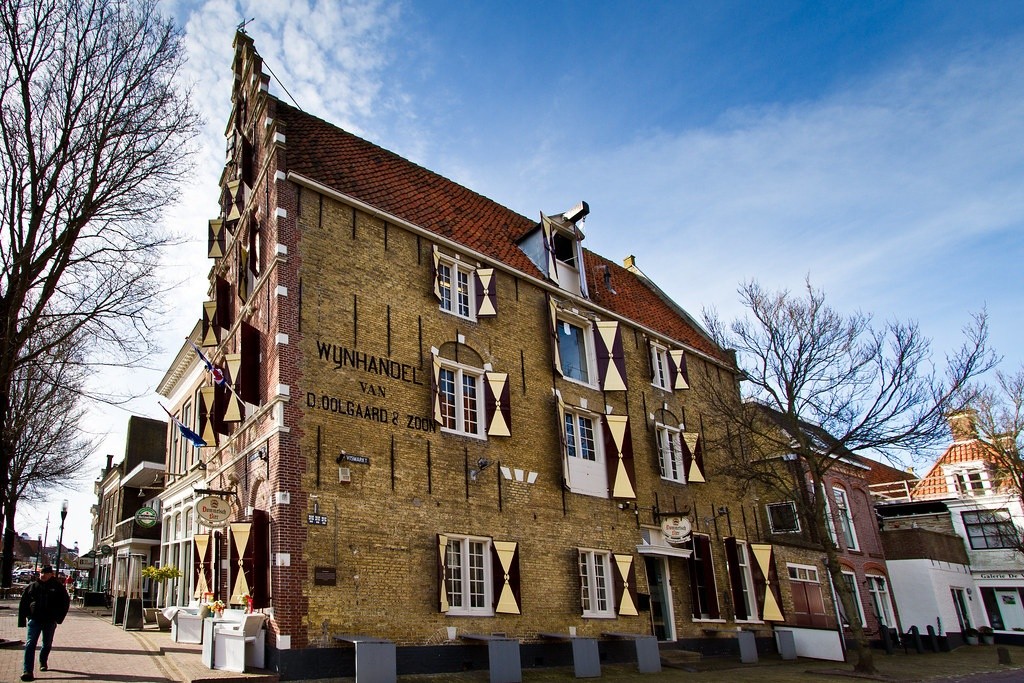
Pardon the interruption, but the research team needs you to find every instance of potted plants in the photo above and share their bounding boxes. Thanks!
[963,627,978,645]
[979,626,994,645]
[141,565,182,631]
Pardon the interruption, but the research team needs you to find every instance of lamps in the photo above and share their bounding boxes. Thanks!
[471,457,494,480]
[154,470,187,483]
[706,506,731,527]
[138,485,169,496]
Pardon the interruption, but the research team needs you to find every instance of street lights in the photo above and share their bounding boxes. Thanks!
[34,533,43,572]
[54,498,69,579]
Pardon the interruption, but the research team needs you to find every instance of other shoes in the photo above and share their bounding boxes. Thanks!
[40,661,47,671]
[21,671,34,681]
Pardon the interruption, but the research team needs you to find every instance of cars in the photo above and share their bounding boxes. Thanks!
[12,570,31,584]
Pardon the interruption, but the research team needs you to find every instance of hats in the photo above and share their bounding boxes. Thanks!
[41,566,52,574]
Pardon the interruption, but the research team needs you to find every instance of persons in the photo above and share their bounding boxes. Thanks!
[18,566,70,681]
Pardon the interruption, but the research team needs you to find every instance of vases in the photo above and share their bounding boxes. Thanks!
[214,610,222,618]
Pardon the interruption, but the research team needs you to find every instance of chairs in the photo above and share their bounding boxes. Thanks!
[162,601,266,673]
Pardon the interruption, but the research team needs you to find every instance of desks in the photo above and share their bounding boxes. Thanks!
[741,627,796,661]
[539,633,601,679]
[601,633,661,674]
[458,634,522,683]
[333,635,396,683]
[701,628,759,664]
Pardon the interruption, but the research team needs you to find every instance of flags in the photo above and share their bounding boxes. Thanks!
[189,340,227,387]
[163,407,207,448]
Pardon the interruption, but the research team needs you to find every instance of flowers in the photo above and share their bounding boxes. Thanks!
[209,600,226,611]
[237,587,259,614]
